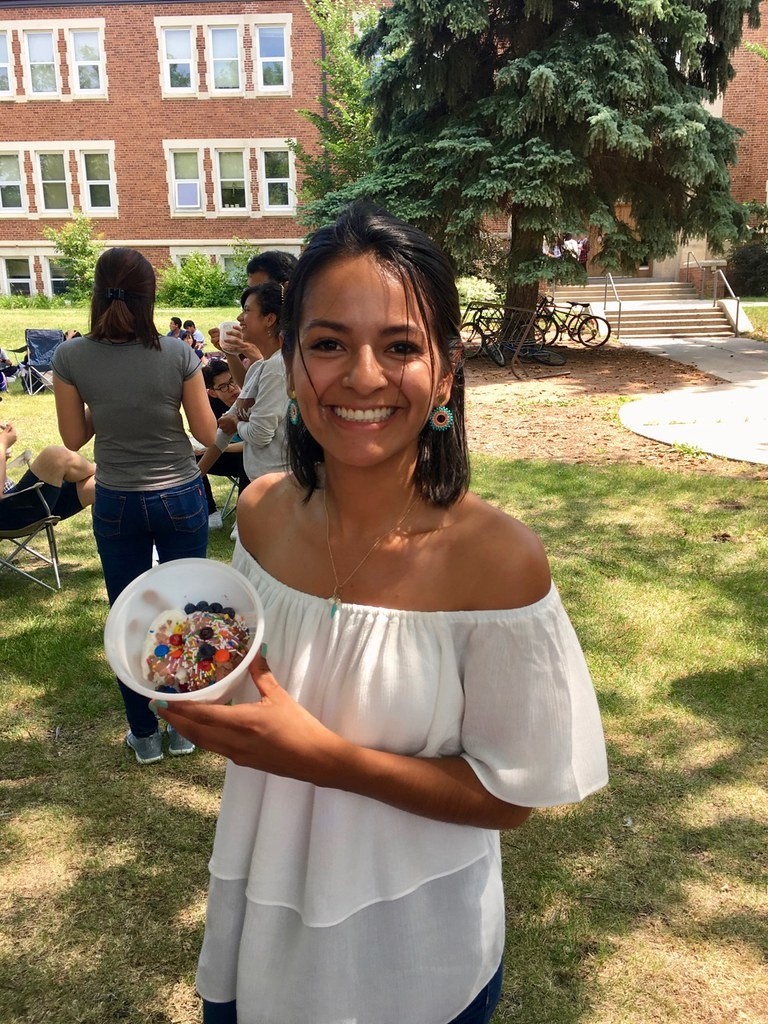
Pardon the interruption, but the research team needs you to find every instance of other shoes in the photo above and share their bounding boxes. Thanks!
[230,515,240,542]
[207,509,223,530]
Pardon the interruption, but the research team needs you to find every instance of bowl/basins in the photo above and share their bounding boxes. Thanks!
[102,557,265,705]
[219,322,242,355]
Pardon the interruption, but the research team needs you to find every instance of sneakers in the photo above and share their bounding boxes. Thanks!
[126,725,163,765]
[166,721,196,757]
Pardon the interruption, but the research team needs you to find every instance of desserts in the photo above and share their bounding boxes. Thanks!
[140,601,251,693]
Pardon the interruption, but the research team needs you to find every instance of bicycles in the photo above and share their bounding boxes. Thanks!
[458,293,611,367]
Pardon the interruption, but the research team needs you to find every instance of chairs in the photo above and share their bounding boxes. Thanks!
[221,476,239,521]
[3,328,65,396]
[0,449,61,593]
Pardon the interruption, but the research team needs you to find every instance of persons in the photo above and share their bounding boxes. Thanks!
[0,348,18,392]
[167,251,300,544]
[64,329,81,340]
[543,232,590,272]
[149,201,609,1024]
[52,248,245,764]
[0,419,95,532]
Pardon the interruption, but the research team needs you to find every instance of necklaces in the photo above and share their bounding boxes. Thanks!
[322,478,424,618]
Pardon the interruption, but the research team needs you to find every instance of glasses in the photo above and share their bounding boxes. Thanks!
[210,376,236,394]
